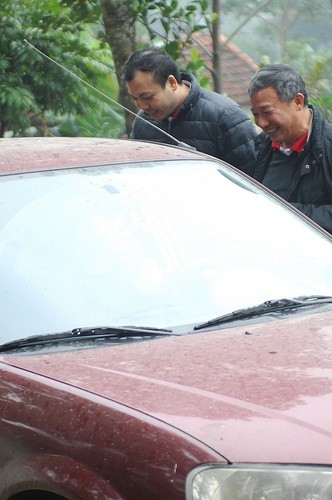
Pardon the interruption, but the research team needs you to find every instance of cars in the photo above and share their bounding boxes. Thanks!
[1,137,331,500]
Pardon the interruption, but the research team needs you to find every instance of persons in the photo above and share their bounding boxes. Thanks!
[247,64,332,236]
[123,48,257,179]
